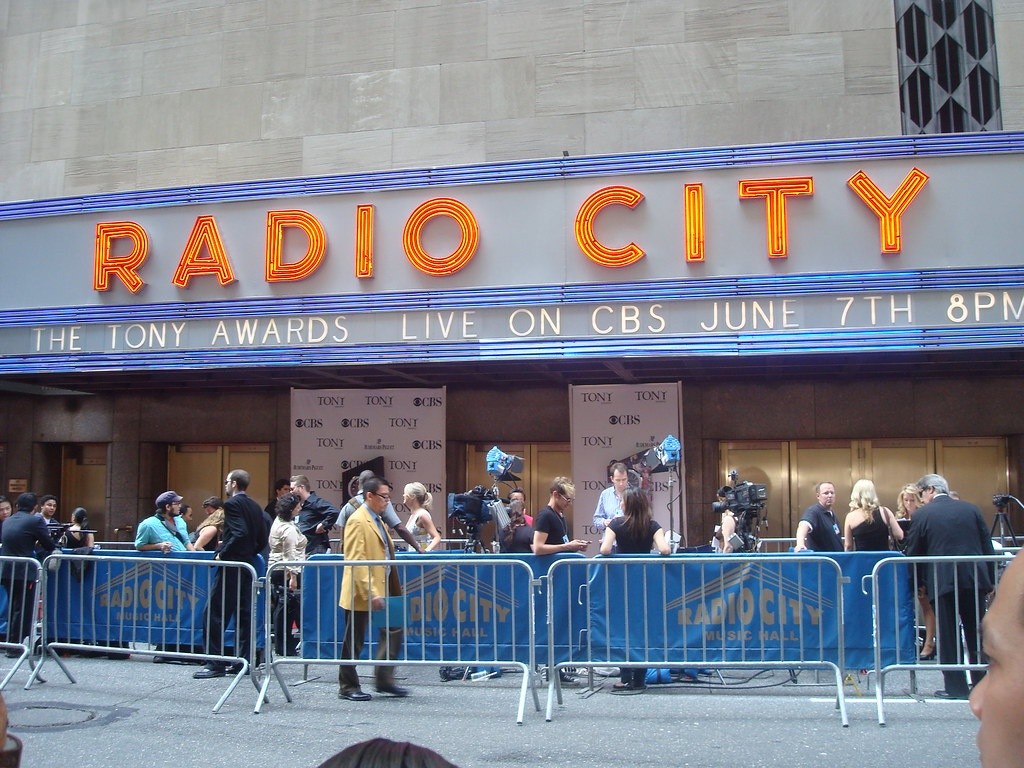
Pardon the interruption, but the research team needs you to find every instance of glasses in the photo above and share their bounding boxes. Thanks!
[918,486,927,498]
[558,492,575,503]
[369,491,390,501]
[225,479,233,485]
[169,501,180,506]
[289,484,302,491]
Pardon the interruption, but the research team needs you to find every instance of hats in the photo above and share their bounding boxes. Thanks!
[155,490,183,508]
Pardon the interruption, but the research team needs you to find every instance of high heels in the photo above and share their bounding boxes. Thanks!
[920,647,936,660]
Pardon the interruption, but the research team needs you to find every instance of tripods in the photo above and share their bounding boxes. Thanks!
[989,494,1024,555]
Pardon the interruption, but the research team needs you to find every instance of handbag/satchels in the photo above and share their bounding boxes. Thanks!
[277,585,301,605]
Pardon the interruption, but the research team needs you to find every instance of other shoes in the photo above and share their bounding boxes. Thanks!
[613,680,645,689]
[0,639,205,665]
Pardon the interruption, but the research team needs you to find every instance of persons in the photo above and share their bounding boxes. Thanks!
[968,545,1024,768]
[599,486,672,691]
[338,476,411,701]
[499,488,538,559]
[593,461,652,557]
[890,483,937,660]
[712,485,740,554]
[905,474,996,700]
[0,493,94,661]
[532,477,592,687]
[793,481,844,554]
[844,479,904,552]
[133,470,340,678]
[333,470,442,572]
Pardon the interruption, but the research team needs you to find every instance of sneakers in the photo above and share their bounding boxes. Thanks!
[545,673,580,686]
[558,666,576,673]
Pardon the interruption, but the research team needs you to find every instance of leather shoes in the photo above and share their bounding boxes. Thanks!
[193,668,226,679]
[338,691,371,701]
[224,665,251,676]
[374,684,410,697]
[935,689,969,700]
